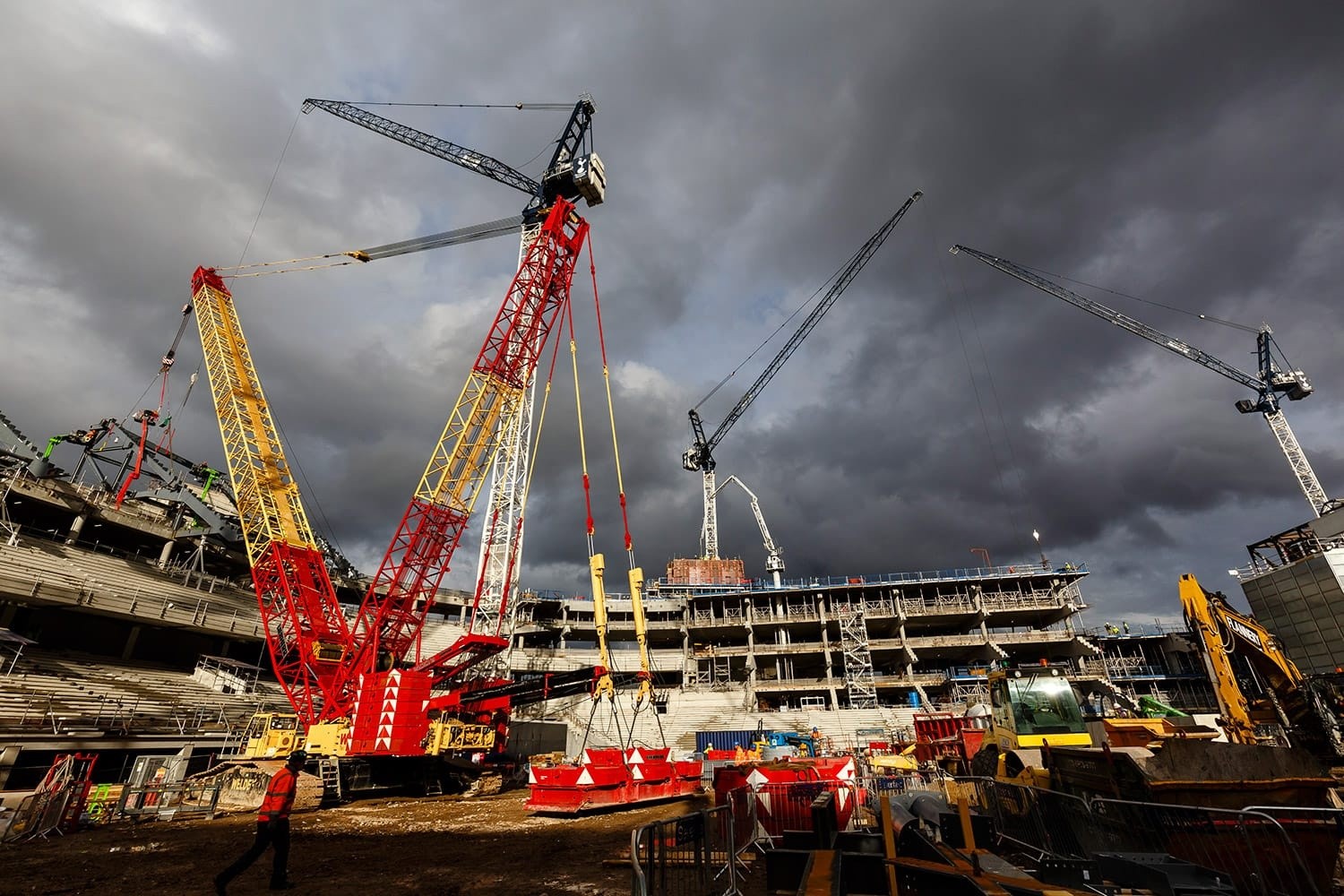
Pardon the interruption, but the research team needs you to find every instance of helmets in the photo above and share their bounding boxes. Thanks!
[708,743,712,746]
[1114,703,1121,709]
[285,751,308,761]
[813,727,817,731]
[752,744,756,748]
[734,742,739,746]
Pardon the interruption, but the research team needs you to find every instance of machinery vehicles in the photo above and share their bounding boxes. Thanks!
[913,712,991,775]
[1176,570,1341,764]
[968,660,1344,896]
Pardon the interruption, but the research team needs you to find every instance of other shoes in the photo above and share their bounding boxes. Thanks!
[213,873,226,896]
[269,881,294,889]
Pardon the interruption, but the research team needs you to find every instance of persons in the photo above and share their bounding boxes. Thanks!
[703,742,713,754]
[734,741,761,767]
[799,726,823,757]
[212,750,308,896]
[1065,561,1072,572]
[1114,703,1128,719]
[1337,696,1344,717]
[1104,621,1129,635]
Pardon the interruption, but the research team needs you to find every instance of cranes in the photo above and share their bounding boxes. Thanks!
[681,189,925,643]
[950,243,1334,518]
[187,94,703,813]
